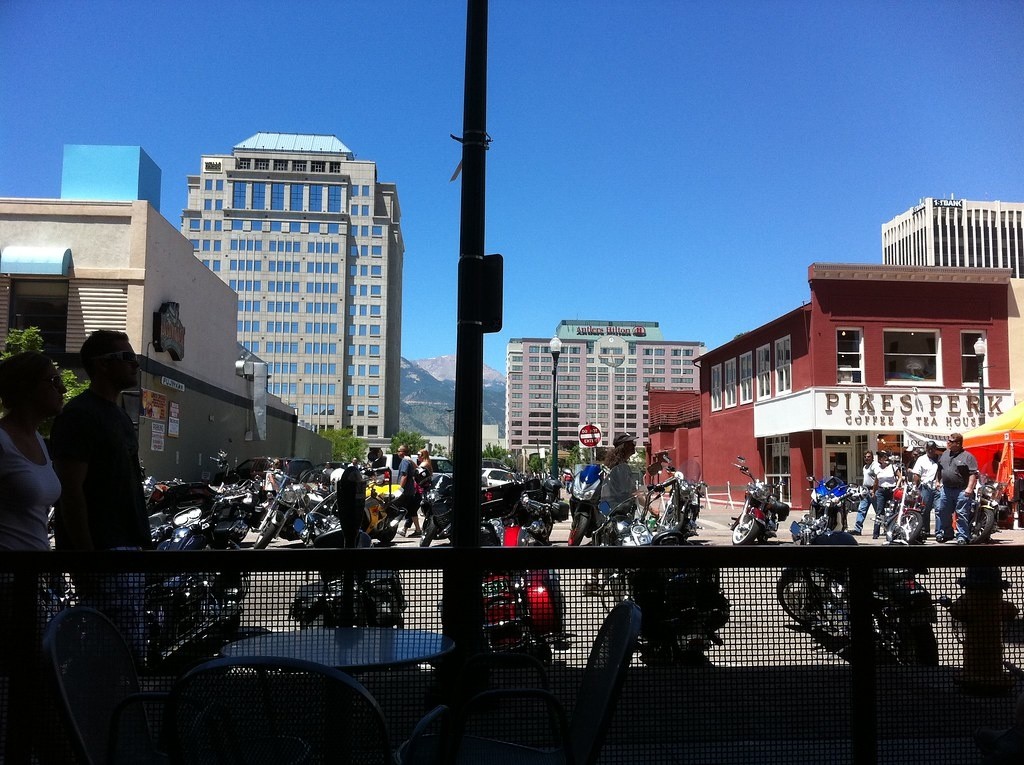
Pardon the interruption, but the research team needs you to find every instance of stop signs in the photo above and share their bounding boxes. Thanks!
[577,424,603,449]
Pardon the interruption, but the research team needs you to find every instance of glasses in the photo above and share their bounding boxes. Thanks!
[397,449,404,453]
[43,375,64,390]
[948,438,957,443]
[417,453,421,457]
[106,350,136,364]
[865,456,870,458]
[626,440,636,446]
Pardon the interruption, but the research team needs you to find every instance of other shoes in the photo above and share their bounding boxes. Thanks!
[848,529,862,536]
[872,536,879,539]
[957,534,969,544]
[936,532,955,543]
[584,581,610,598]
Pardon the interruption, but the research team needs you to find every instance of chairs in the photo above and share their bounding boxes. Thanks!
[42,606,200,765]
[159,657,452,765]
[396,601,643,764]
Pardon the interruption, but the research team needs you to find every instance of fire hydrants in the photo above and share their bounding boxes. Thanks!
[947,566,1021,673]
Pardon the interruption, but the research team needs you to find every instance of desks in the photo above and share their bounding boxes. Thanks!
[220,628,455,765]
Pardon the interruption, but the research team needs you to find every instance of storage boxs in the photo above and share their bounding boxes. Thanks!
[314,530,370,582]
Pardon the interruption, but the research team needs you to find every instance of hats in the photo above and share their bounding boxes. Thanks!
[927,441,937,449]
[876,449,889,455]
[613,433,640,448]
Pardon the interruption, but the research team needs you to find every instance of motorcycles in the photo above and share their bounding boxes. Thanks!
[774,461,1019,667]
[726,454,790,546]
[43,448,731,667]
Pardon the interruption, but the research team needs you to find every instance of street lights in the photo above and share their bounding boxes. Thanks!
[445,408,454,455]
[549,335,563,480]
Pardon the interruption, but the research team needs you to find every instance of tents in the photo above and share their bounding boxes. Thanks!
[947,398,1024,530]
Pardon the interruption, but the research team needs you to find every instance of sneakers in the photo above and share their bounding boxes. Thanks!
[397,528,407,538]
[408,531,424,538]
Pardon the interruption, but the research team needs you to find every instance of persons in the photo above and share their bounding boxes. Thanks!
[50,329,153,765]
[0,352,67,764]
[907,450,922,481]
[563,472,573,494]
[396,444,433,537]
[330,462,346,489]
[582,431,660,595]
[911,440,943,541]
[936,433,977,545]
[265,458,282,497]
[322,462,334,476]
[847,447,880,535]
[872,449,902,539]
[348,458,361,470]
